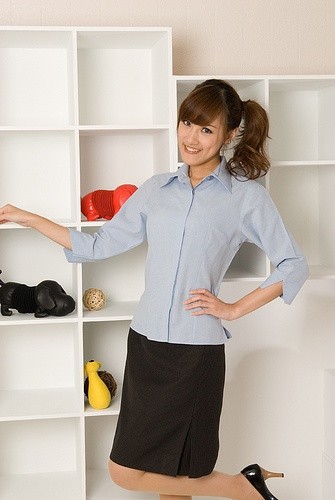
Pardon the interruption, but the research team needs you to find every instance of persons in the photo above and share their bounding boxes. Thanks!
[0,79,309,500]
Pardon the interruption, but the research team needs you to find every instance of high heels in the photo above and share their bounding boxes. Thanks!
[240,463,284,500]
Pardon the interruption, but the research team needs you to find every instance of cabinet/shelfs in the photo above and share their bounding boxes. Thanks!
[0,26,174,500]
[173,74,334,281]
